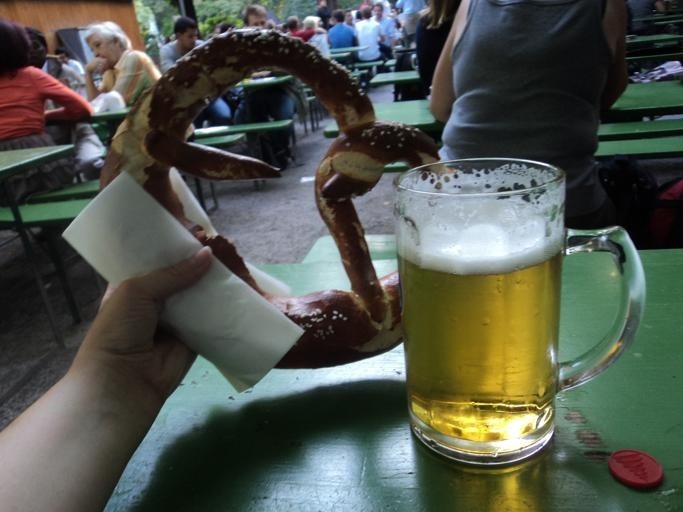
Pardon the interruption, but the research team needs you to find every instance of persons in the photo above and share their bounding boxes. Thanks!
[2,17,91,205]
[303,1,427,75]
[159,17,232,128]
[426,1,627,229]
[0,246,214,512]
[417,1,461,98]
[85,21,195,143]
[50,48,89,100]
[212,4,310,171]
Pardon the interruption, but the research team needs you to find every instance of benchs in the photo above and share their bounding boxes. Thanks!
[0,12,683,321]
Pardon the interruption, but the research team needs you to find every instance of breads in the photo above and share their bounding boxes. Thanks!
[100,23,457,368]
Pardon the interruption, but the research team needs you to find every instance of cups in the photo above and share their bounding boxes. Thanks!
[392,156,645,469]
